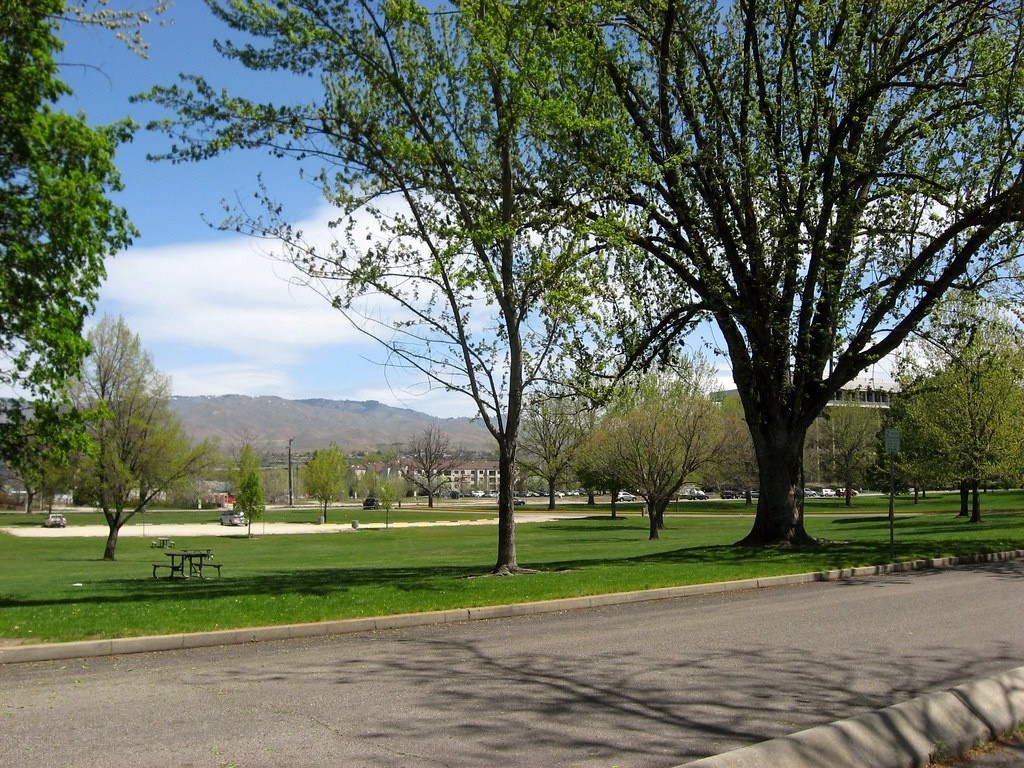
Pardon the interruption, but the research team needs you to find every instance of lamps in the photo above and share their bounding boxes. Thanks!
[211,554,213,560]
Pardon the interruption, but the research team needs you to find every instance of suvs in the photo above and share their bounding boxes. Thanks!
[219,509,249,526]
[617,492,637,502]
[836,487,859,497]
[362,497,380,509]
[804,487,817,498]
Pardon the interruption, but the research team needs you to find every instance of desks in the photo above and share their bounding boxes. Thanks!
[153,552,221,582]
[180,549,213,560]
[152,538,174,549]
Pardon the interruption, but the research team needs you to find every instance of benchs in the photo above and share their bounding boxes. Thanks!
[169,541,175,544]
[152,563,181,569]
[151,541,157,544]
[192,562,222,568]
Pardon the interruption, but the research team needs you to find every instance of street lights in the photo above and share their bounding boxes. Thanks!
[286,436,295,505]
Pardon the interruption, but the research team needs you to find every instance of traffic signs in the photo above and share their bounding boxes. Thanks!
[885,430,900,451]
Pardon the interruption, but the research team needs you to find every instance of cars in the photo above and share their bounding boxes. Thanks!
[512,497,525,506]
[44,514,67,528]
[679,485,760,500]
[432,486,613,498]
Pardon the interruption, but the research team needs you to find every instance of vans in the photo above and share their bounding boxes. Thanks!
[822,488,836,497]
